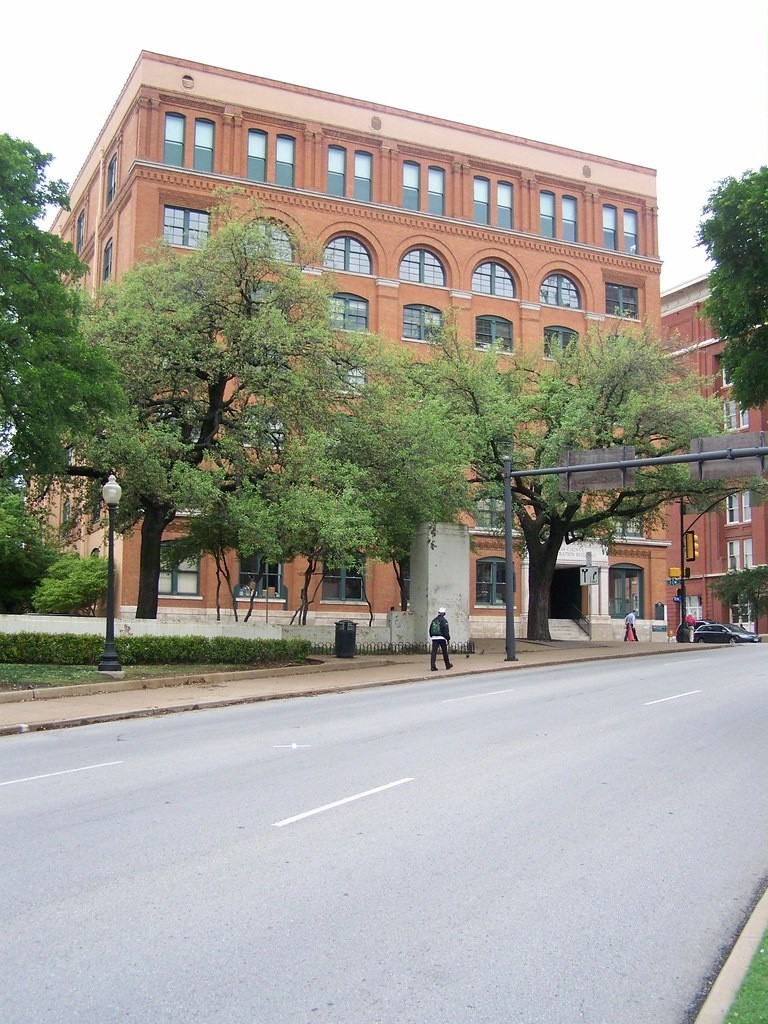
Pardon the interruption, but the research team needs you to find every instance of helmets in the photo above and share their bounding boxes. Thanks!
[438,608,446,615]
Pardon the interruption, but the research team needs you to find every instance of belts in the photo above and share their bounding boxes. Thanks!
[688,625,693,626]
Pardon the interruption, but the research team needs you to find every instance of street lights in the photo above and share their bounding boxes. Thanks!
[96,474,122,670]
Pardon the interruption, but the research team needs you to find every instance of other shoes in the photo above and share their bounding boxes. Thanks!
[431,667,438,671]
[446,664,453,670]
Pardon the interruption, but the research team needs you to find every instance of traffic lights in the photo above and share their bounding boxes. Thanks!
[684,567,691,578]
[685,529,700,562]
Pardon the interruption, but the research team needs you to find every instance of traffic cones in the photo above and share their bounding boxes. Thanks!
[627,625,637,641]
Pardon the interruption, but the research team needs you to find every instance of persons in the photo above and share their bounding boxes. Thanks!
[429,607,453,671]
[687,613,696,628]
[624,610,638,642]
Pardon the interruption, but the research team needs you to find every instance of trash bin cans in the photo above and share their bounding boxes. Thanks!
[333,620,358,658]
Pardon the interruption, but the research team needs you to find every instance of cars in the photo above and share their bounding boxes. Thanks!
[694,622,761,643]
[675,618,720,643]
